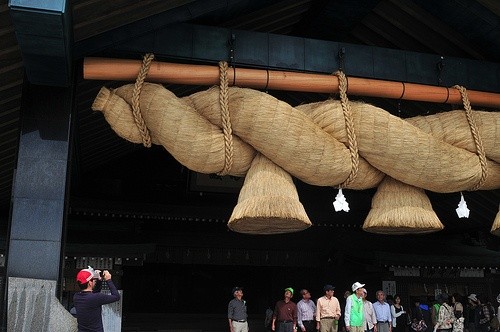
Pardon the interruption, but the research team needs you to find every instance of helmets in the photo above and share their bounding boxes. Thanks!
[285,288,293,294]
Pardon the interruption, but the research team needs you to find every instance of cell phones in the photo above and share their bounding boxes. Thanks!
[99,272,105,277]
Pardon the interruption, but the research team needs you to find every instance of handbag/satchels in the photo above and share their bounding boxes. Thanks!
[411,320,427,331]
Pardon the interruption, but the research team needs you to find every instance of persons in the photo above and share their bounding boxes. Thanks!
[296,289,317,332]
[228,287,250,332]
[72,269,120,332]
[316,285,342,332]
[271,287,298,332]
[340,281,500,332]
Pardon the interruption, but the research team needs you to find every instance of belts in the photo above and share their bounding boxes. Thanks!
[277,319,293,323]
[320,317,335,319]
[233,319,246,322]
[377,320,388,323]
[303,320,315,323]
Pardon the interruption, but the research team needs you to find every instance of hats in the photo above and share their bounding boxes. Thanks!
[77,269,99,284]
[468,294,477,301]
[363,288,367,293]
[232,287,241,292]
[324,285,335,291]
[352,282,365,292]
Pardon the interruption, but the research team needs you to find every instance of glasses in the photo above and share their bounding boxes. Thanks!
[303,291,309,295]
